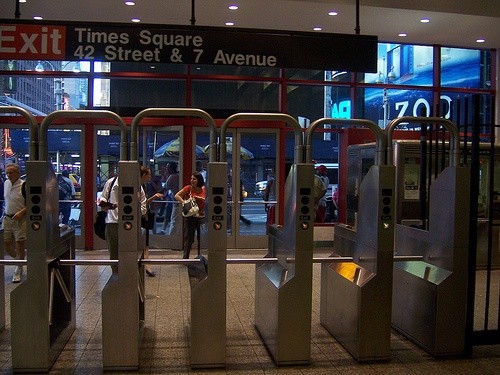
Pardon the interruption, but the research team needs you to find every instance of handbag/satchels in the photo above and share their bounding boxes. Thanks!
[59,189,66,200]
[141,212,156,229]
[182,186,199,217]
[94,211,108,240]
[188,255,208,278]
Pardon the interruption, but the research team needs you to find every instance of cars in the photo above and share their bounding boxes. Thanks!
[57,173,81,199]
[255,181,268,199]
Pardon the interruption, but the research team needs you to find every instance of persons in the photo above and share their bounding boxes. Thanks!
[174,173,208,260]
[50,156,342,236]
[2,164,27,282]
[99,174,147,276]
[137,166,165,276]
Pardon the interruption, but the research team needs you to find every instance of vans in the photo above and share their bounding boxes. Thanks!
[313,164,339,220]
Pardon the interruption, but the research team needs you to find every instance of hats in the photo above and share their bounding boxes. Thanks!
[317,165,327,174]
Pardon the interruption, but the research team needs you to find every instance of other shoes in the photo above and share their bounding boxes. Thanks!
[12,273,21,281]
[184,262,190,266]
[146,269,157,275]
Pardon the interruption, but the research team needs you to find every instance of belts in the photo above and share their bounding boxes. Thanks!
[5,214,14,218]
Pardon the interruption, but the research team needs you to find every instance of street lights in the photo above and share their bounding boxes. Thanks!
[376,66,398,130]
